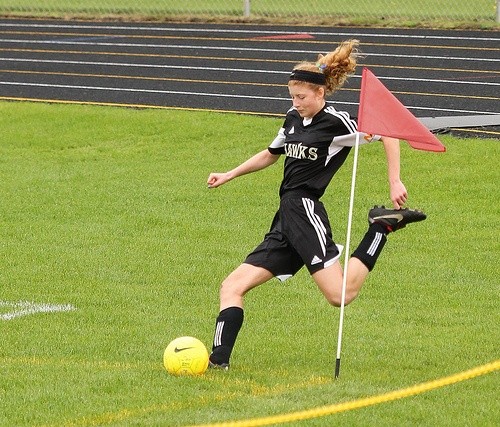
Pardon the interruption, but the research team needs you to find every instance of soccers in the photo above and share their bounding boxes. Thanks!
[163,336,209,377]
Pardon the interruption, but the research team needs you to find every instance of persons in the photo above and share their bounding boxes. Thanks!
[205,40,428,371]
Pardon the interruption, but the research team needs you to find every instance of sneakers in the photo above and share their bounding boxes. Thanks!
[207,361,230,372]
[368,205,426,228]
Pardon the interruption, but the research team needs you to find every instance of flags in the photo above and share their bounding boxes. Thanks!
[358,67,446,153]
